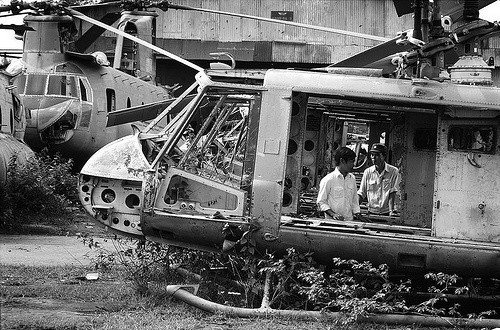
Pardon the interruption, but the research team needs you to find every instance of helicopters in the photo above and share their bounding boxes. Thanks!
[0,0,500,330]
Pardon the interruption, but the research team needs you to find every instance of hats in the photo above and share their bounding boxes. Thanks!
[370,143,389,155]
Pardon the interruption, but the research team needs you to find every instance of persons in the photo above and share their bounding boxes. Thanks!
[317,146,371,223]
[356,144,401,217]
